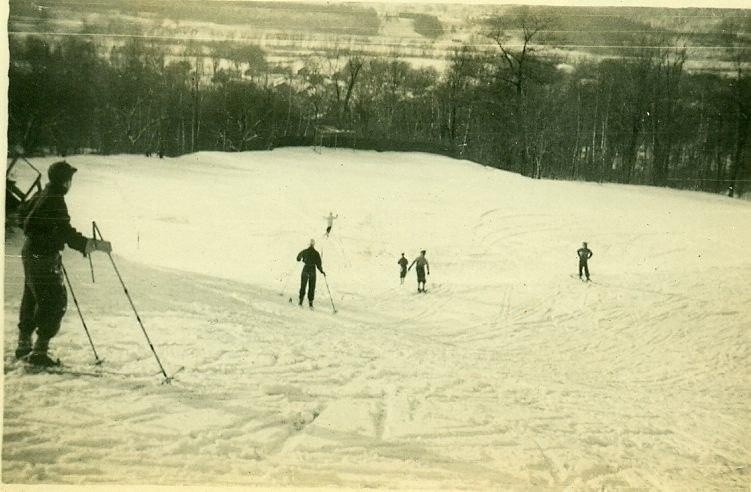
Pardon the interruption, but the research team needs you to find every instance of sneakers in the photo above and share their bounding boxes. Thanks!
[27,349,54,367]
[15,346,32,360]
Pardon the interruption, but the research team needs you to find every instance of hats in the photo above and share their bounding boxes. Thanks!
[47,162,77,180]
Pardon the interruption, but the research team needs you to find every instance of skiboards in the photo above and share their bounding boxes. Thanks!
[4,357,184,385]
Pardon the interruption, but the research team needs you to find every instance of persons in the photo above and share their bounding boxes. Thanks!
[15,161,112,368]
[296,239,325,307]
[408,250,429,292]
[577,242,593,280]
[323,212,338,238]
[398,253,408,284]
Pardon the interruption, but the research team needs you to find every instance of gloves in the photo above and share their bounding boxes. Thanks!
[96,239,111,254]
